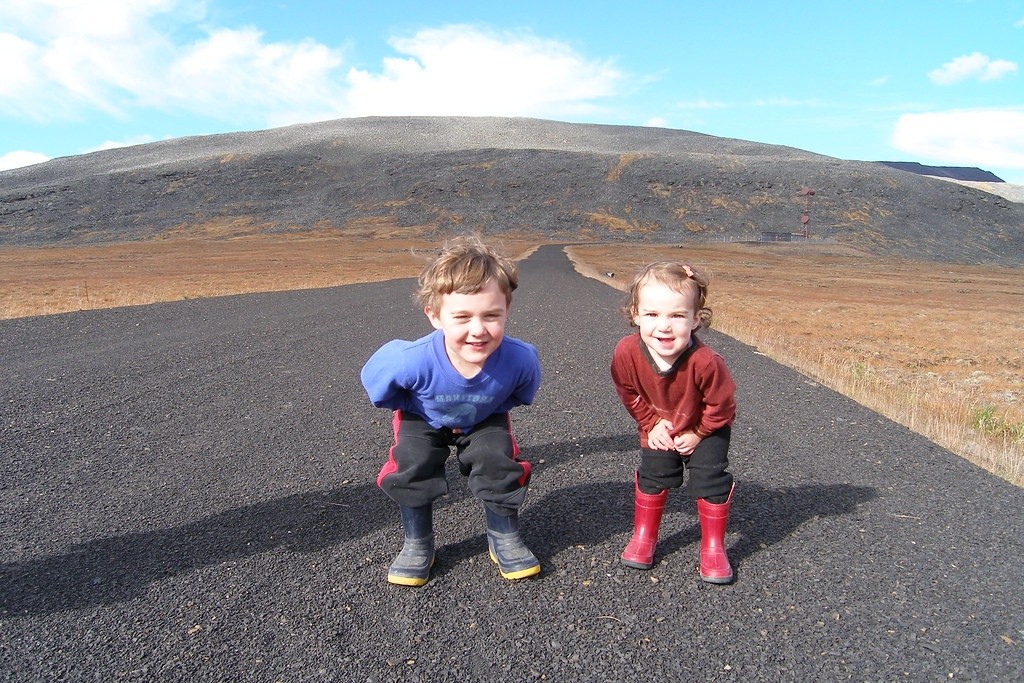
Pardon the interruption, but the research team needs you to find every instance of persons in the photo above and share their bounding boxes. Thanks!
[359,236,542,587]
[609,258,737,585]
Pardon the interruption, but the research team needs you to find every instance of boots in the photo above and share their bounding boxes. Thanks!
[388,503,435,585]
[483,502,541,580]
[698,482,736,584]
[621,472,670,569]
